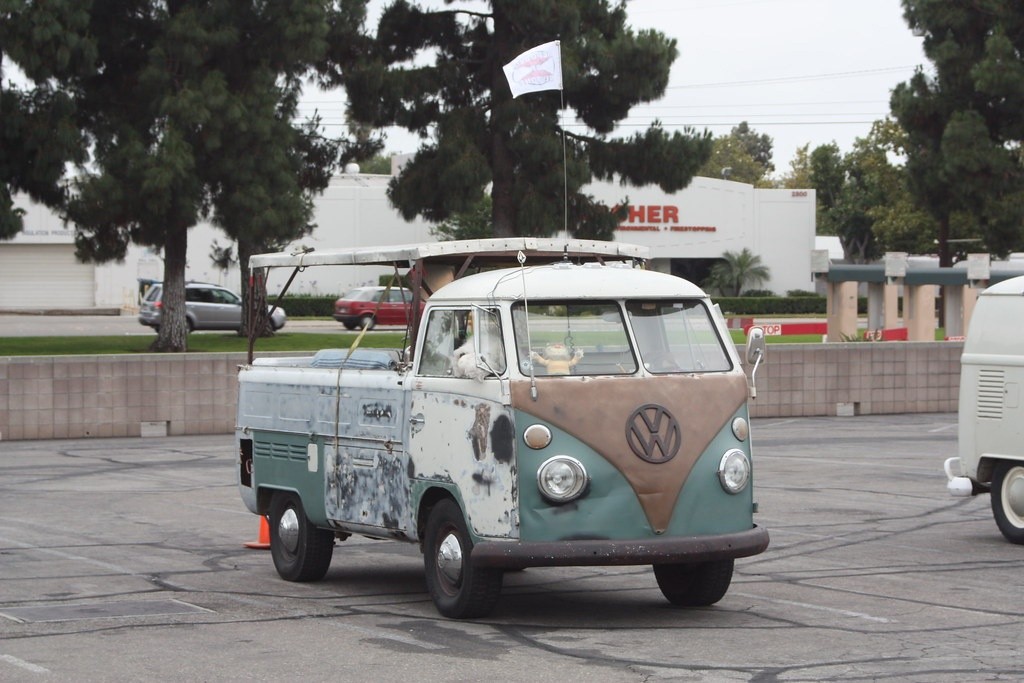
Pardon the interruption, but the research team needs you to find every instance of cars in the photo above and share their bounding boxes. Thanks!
[334,288,430,332]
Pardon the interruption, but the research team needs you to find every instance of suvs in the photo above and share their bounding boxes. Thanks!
[139,283,286,333]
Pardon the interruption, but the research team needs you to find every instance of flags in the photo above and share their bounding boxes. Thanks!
[502,40,564,99]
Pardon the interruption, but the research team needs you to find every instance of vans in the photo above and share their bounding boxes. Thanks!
[943,271,1024,542]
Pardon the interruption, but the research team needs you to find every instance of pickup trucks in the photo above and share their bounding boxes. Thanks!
[235,239,772,617]
